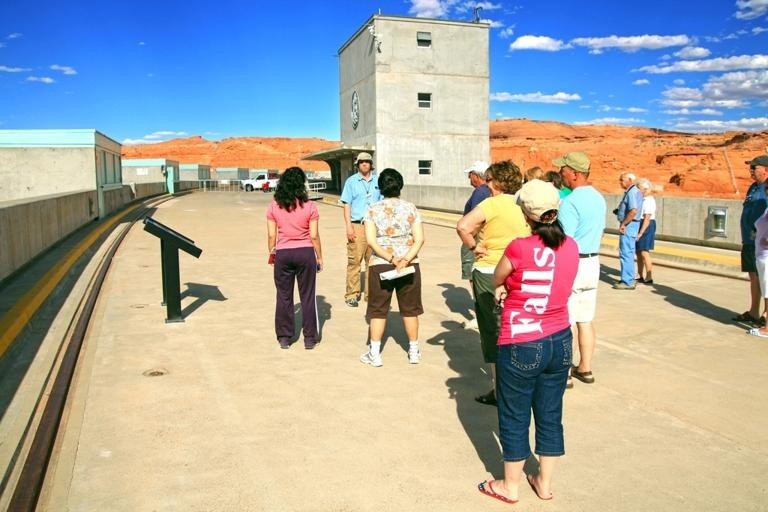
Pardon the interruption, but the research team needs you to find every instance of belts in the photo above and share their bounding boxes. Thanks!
[351,221,362,223]
[579,253,597,257]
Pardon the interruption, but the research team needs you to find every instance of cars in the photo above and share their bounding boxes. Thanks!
[304,171,322,179]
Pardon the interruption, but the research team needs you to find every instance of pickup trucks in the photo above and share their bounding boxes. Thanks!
[240,171,281,192]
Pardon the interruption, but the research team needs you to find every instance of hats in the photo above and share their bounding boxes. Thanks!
[745,155,767,166]
[353,152,374,170]
[464,160,489,174]
[552,151,592,174]
[515,178,560,224]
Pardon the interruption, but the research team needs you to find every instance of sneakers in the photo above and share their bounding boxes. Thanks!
[749,328,767,337]
[613,282,636,289]
[345,298,358,307]
[408,348,421,364]
[359,351,383,367]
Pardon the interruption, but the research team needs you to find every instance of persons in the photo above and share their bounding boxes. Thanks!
[742,179,767,338]
[612,172,644,290]
[481,180,580,505]
[547,150,606,388]
[339,153,384,308]
[733,156,767,324]
[264,167,324,351]
[358,167,425,367]
[455,160,532,408]
[633,177,657,286]
[460,148,573,332]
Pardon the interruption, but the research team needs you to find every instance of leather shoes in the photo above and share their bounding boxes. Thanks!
[638,279,653,285]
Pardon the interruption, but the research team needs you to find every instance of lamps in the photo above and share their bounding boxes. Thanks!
[706,205,730,239]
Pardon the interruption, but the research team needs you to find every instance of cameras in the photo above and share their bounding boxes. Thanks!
[612,208,619,215]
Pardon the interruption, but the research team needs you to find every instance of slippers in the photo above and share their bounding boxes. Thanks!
[474,389,499,406]
[732,311,753,321]
[477,479,519,503]
[527,472,553,500]
[751,317,766,328]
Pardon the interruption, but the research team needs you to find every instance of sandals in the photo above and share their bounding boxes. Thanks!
[571,366,594,384]
[565,376,574,387]
